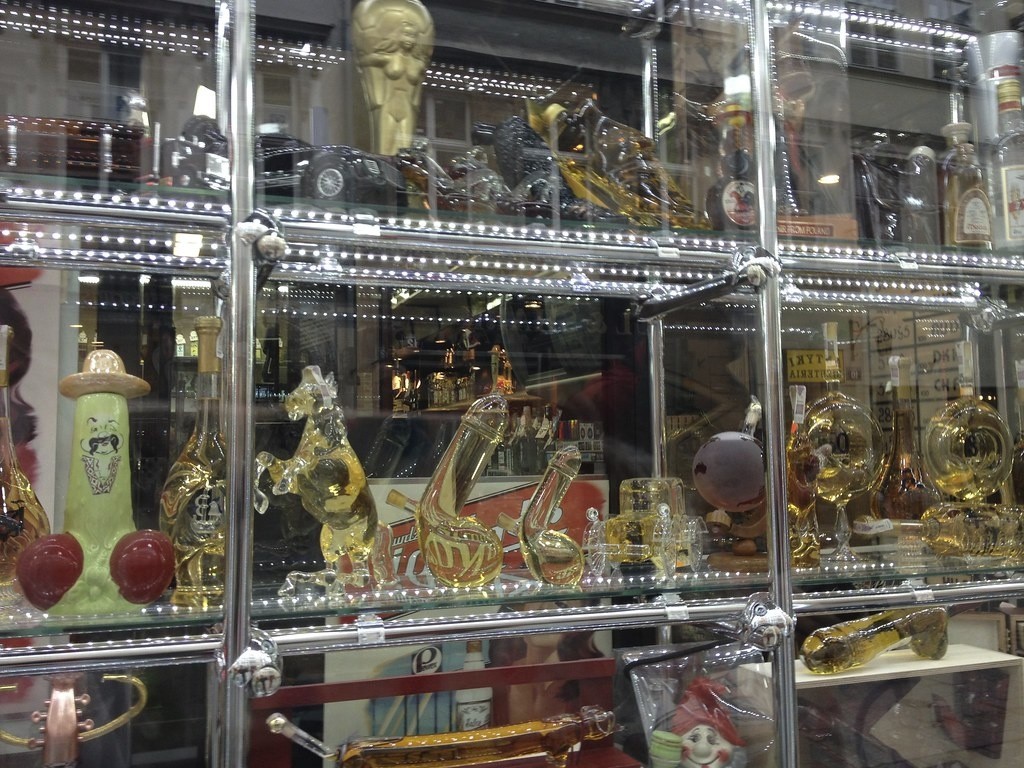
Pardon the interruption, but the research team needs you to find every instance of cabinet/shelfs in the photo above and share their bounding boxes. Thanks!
[0,0,1024,768]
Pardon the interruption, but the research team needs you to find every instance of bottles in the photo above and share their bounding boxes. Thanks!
[1,327,52,608]
[869,356,942,535]
[707,104,762,234]
[989,62,1023,252]
[160,314,227,605]
[782,384,820,568]
[456,640,494,730]
[937,123,993,253]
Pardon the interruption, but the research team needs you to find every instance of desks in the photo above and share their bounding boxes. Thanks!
[736,643,1024,768]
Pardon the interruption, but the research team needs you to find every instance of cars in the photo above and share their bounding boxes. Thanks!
[171,131,410,207]
